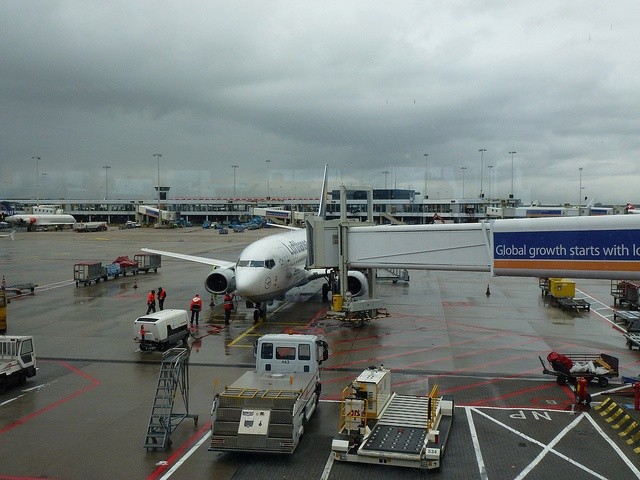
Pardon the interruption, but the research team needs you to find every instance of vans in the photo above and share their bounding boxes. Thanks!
[211,222,218,228]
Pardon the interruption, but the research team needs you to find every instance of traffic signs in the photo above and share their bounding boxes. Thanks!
[508,152,517,199]
[152,152,165,229]
[488,166,493,201]
[478,149,487,200]
[265,160,272,200]
[31,155,41,212]
[231,165,240,202]
[103,165,112,200]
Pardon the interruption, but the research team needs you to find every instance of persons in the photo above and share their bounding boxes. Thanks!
[146,290,156,316]
[224,289,234,325]
[157,286,167,310]
[190,293,201,325]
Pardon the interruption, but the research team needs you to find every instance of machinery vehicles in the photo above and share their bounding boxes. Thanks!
[133,252,161,274]
[73,261,107,287]
[207,328,330,455]
[0,335,37,388]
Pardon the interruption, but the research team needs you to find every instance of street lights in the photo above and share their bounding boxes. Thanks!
[579,168,585,205]
[423,153,429,202]
[381,172,390,195]
[460,167,467,202]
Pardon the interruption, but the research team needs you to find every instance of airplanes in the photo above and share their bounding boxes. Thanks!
[139,162,369,323]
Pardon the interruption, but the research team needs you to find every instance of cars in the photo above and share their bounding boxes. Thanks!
[228,224,235,228]
[202,222,210,229]
[233,225,244,232]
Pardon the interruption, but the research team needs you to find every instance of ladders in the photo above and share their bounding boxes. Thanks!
[143,348,198,453]
[376,268,410,284]
